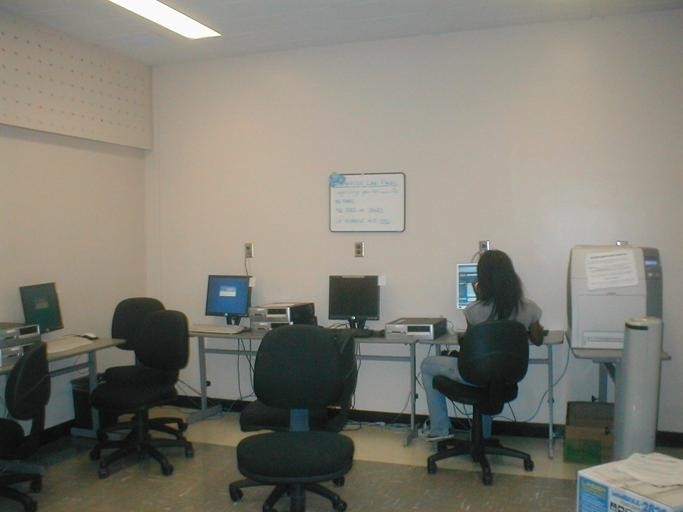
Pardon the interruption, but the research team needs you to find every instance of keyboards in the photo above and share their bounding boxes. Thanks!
[189,323,243,334]
[46,335,93,353]
[324,328,373,337]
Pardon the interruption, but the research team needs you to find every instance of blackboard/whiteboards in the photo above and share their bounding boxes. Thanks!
[330,173,405,231]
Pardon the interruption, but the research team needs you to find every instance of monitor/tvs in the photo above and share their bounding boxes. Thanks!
[329,275,382,320]
[205,275,253,317]
[456,263,477,309]
[20,282,64,333]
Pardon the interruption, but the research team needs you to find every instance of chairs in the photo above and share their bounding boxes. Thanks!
[240,397,351,436]
[227,326,355,511]
[98,295,188,437]
[425,320,535,487]
[87,311,195,479]
[0,341,56,511]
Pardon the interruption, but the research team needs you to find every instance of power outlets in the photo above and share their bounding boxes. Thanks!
[354,240,365,258]
[245,243,253,257]
[479,240,489,258]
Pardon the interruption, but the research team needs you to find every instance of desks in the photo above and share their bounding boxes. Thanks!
[0,336,126,447]
[183,330,565,459]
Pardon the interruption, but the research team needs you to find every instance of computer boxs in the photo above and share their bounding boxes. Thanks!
[248,302,315,322]
[250,316,317,333]
[384,317,447,340]
[0,322,40,348]
[0,343,36,368]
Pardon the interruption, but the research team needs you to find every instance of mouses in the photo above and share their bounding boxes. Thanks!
[79,332,97,339]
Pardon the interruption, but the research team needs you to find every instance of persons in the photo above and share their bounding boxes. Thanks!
[420,249,545,442]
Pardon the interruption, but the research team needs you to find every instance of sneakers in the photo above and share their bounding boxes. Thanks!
[422,431,455,442]
